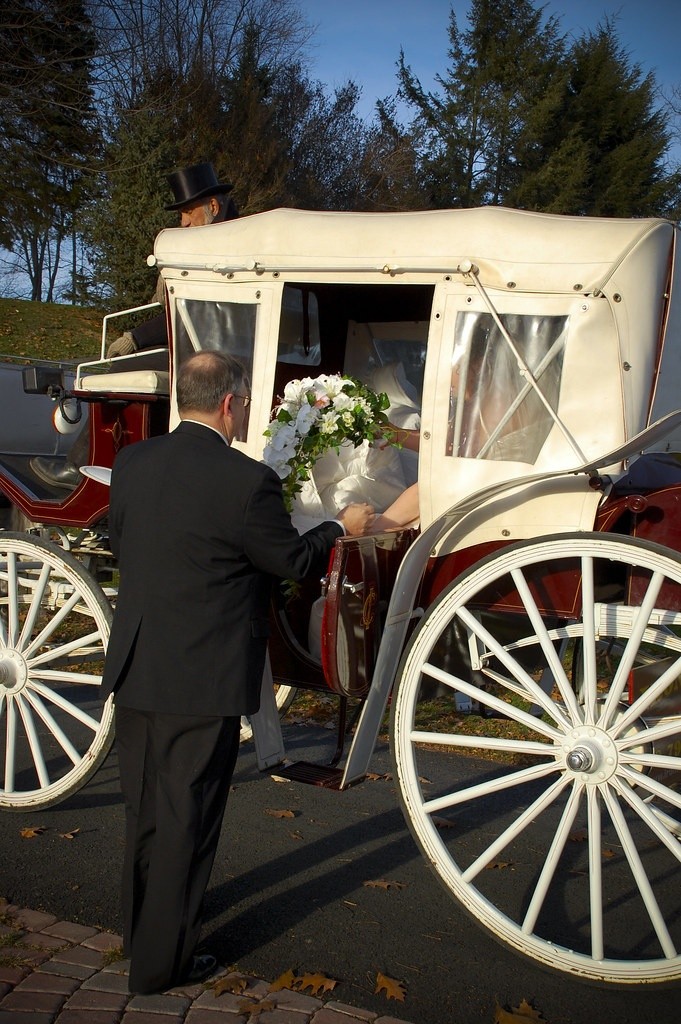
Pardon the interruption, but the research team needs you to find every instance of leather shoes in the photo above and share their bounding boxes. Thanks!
[178,955,219,986]
[30,456,85,490]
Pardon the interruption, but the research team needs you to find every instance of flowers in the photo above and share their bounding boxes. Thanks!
[258,370,409,518]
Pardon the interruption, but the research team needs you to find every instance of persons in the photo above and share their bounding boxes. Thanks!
[290,329,556,666]
[28,160,241,490]
[99,351,376,997]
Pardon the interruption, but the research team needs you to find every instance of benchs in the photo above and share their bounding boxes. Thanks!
[74,303,172,402]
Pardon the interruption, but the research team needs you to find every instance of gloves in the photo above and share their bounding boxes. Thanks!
[152,274,164,303]
[106,332,138,366]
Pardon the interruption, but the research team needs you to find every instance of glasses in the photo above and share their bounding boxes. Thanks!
[222,395,252,407]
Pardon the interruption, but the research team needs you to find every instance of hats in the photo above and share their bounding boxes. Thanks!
[164,164,235,210]
